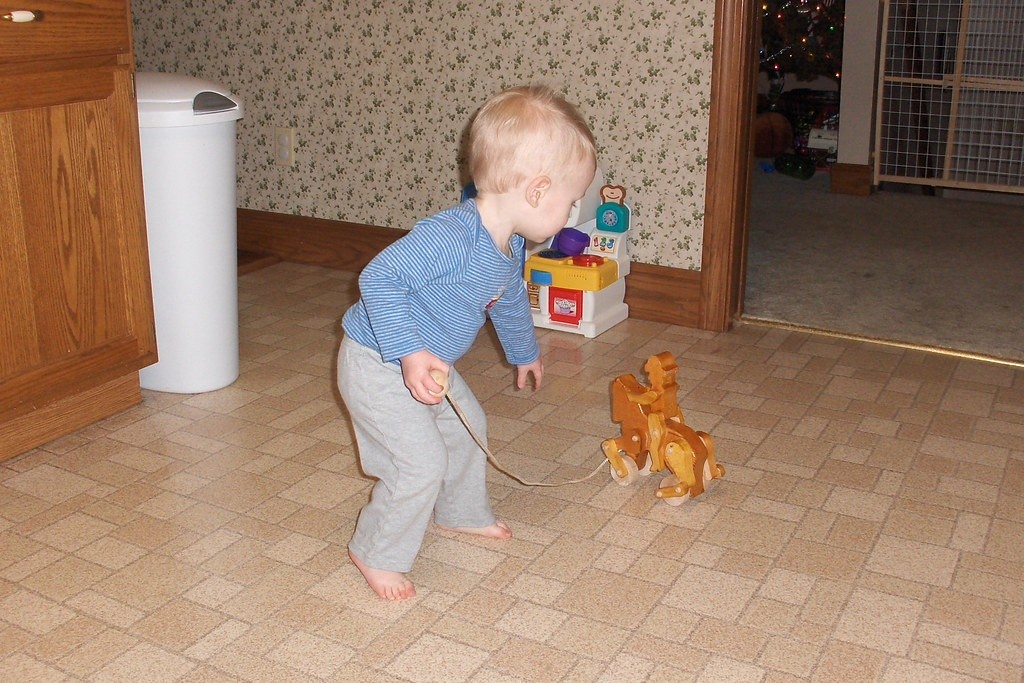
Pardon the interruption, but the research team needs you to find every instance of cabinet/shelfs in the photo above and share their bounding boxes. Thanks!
[0,0,158,463]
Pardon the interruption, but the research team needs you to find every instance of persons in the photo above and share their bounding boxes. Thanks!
[337,78,597,601]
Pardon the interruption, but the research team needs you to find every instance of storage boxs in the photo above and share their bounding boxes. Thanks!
[808,127,839,150]
[831,163,874,196]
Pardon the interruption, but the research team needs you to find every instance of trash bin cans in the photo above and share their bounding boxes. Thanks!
[135,72,247,394]
